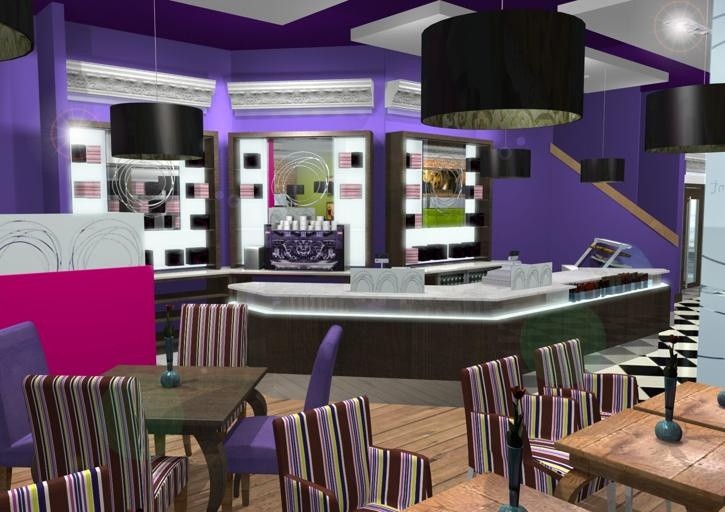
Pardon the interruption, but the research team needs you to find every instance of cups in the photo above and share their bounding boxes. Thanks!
[276,215,337,231]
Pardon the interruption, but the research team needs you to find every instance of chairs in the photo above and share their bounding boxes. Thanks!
[19,371,191,511]
[419,1,588,130]
[269,392,435,512]
[533,336,636,425]
[459,351,580,498]
[176,305,252,454]
[473,143,535,181]
[219,321,347,512]
[639,84,723,158]
[574,158,631,184]
[1,316,54,461]
[1,462,113,511]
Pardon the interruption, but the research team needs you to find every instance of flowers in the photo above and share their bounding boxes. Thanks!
[661,334,679,376]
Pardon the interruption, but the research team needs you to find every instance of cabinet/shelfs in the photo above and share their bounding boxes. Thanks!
[67,122,493,270]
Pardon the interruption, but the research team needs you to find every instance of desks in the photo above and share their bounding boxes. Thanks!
[380,466,602,512]
[554,381,725,512]
[90,360,269,512]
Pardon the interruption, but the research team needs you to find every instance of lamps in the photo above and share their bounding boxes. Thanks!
[109,1,206,163]
[1,0,33,62]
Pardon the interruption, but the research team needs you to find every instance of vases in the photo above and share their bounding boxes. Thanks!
[655,379,681,441]
